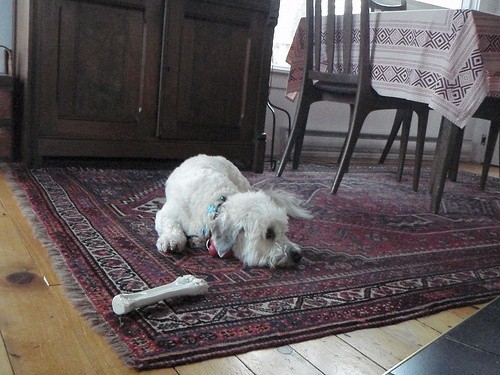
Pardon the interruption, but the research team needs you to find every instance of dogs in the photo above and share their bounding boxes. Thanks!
[155,152,313,269]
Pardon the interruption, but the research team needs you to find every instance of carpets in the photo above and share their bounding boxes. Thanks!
[5,162,500,375]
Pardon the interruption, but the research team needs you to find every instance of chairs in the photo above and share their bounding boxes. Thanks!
[281,0,500,195]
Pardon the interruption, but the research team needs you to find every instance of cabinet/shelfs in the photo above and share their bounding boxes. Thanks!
[12,0,280,174]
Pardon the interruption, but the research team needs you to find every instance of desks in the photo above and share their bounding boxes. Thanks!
[285,9,500,214]
